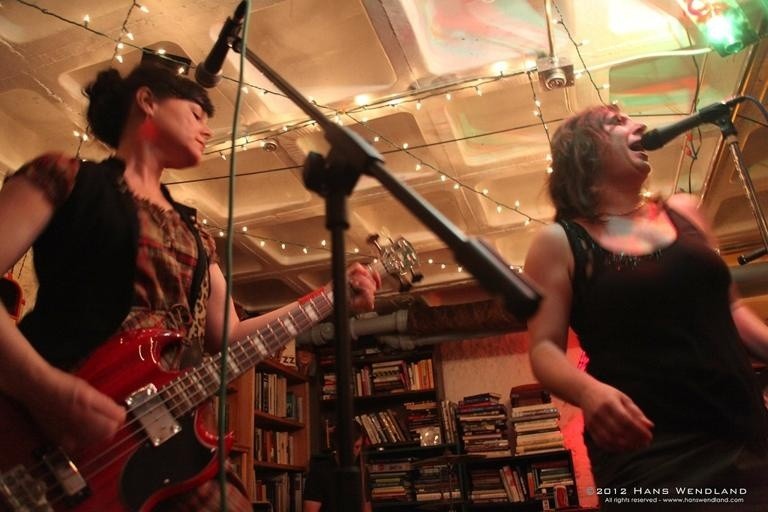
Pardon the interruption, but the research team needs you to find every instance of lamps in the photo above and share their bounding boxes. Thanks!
[677,0,767,58]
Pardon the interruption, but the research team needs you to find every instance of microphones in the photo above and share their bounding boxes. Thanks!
[737,245,768,265]
[640,102,731,151]
[194,14,244,89]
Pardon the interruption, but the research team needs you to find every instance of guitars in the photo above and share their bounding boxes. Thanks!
[0,235,419,510]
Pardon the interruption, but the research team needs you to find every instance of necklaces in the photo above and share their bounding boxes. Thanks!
[593,201,648,222]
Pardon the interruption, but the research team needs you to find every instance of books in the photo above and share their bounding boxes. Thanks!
[255,332,573,509]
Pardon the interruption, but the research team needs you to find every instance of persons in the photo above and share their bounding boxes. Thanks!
[0,56,374,510]
[528,102,766,508]
